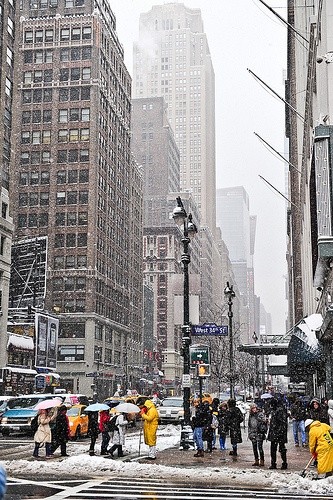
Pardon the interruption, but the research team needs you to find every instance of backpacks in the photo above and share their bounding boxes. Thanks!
[211,414,219,429]
[105,415,119,432]
[31,415,39,432]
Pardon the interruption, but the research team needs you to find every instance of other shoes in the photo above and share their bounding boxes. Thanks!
[61,454,70,457]
[221,446,228,450]
[89,452,95,456]
[269,465,276,469]
[194,451,204,457]
[145,457,156,460]
[204,449,212,453]
[303,443,305,447]
[49,452,54,455]
[100,452,109,455]
[281,465,287,470]
[295,443,299,447]
[212,447,217,450]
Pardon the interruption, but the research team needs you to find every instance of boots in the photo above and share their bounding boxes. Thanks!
[259,458,264,467]
[252,459,259,466]
[117,444,126,458]
[107,444,118,458]
[230,449,237,455]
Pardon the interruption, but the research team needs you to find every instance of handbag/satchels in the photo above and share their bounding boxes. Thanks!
[236,408,244,423]
[258,420,268,433]
[202,429,214,442]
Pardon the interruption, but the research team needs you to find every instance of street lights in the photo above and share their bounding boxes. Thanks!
[251,331,258,396]
[171,196,198,451]
[223,280,236,399]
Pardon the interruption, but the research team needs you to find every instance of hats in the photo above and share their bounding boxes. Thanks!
[305,419,313,428]
[250,403,257,410]
[310,398,321,404]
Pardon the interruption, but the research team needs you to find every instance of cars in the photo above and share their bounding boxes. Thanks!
[0,394,86,437]
[191,390,253,407]
[155,397,196,425]
[101,390,164,420]
[63,404,88,441]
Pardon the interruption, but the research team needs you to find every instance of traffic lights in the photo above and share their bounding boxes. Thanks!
[197,363,211,377]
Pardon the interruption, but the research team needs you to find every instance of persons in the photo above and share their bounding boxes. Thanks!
[300,418,333,481]
[48,405,71,456]
[88,409,128,457]
[189,391,333,469]
[0,460,8,500]
[31,407,58,459]
[140,400,158,460]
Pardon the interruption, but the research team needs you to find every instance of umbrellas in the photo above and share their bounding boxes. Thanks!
[84,402,110,411]
[260,393,275,398]
[34,399,62,414]
[108,403,141,422]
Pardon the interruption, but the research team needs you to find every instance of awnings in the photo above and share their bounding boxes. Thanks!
[287,313,322,364]
